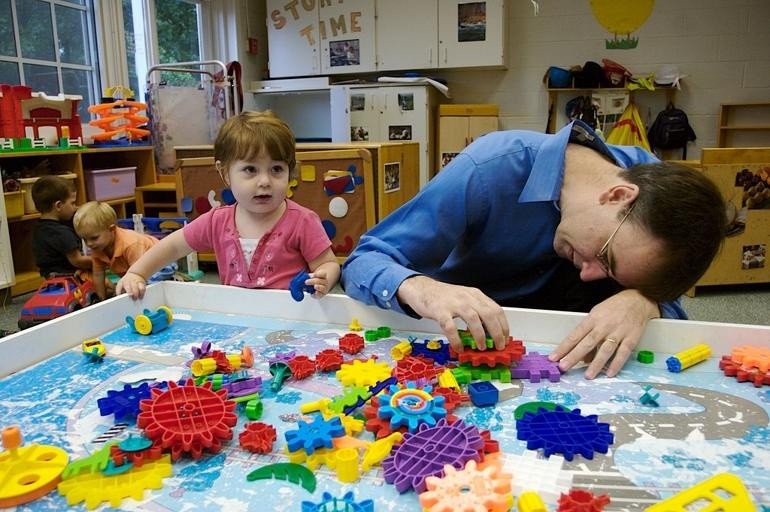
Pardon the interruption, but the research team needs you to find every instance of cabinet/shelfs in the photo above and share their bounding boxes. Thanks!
[716,100,770,147]
[266,0,508,78]
[329,85,435,193]
[436,103,500,175]
[172,140,420,266]
[0,145,179,307]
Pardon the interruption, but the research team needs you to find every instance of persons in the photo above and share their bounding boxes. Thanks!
[462,15,482,26]
[357,125,366,139]
[400,130,409,140]
[340,114,728,383]
[72,199,162,301]
[330,42,357,65]
[29,174,93,279]
[401,97,410,109]
[111,109,340,303]
[385,167,400,189]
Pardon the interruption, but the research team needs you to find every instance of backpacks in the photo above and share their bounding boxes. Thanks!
[648,102,696,151]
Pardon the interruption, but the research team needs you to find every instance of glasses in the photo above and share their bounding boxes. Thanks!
[596,199,638,273]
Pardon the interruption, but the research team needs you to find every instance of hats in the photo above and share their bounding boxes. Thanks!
[655,65,688,84]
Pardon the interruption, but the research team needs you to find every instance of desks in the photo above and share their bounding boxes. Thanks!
[0,280,769,512]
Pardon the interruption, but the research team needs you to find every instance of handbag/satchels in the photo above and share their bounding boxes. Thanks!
[566,96,604,143]
[543,65,572,88]
[573,61,605,88]
[602,59,632,88]
